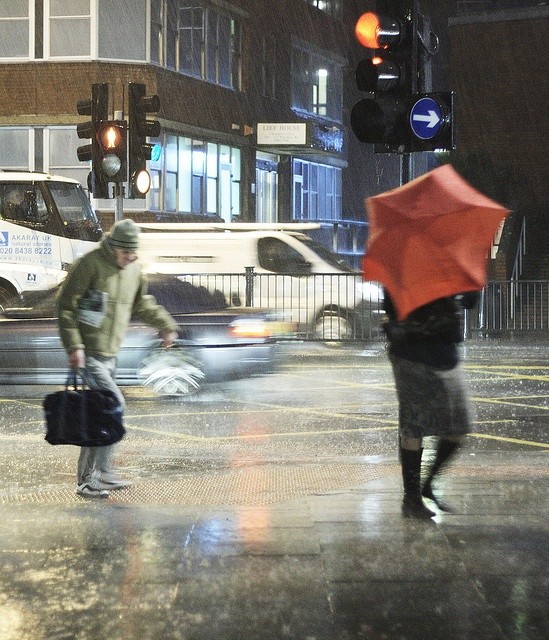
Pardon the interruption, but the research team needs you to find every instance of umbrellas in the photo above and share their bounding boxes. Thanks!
[359,164,511,323]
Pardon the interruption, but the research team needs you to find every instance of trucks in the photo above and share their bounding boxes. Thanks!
[0,168,103,316]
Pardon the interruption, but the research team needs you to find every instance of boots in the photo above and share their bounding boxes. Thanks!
[421,439,461,511]
[398,445,436,517]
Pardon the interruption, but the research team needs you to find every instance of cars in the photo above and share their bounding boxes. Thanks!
[0,270,287,398]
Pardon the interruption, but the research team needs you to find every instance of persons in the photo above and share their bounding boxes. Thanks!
[54,219,180,498]
[381,284,467,520]
[4,190,28,219]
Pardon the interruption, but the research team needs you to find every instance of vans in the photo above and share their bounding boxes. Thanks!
[133,221,388,348]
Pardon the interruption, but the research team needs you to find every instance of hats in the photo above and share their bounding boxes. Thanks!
[108,219,139,251]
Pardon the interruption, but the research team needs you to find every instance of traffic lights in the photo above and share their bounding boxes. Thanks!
[350,1,422,153]
[76,82,113,196]
[128,82,160,199]
[93,120,126,183]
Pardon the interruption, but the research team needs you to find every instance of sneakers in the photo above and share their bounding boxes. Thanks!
[76,483,110,498]
[97,478,132,490]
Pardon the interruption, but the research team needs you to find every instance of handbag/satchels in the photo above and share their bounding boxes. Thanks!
[42,366,126,447]
[382,312,458,371]
